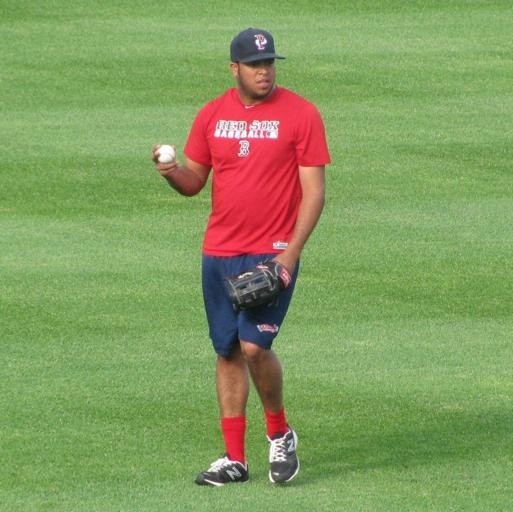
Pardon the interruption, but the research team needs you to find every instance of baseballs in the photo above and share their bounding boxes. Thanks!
[157,145,176,163]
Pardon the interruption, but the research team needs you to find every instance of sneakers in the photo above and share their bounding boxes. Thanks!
[266,422,299,485]
[194,453,249,487]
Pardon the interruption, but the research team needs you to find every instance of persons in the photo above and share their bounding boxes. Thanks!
[151,28,331,485]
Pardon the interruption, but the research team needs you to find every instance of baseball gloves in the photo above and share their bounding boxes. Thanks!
[222,262,293,312]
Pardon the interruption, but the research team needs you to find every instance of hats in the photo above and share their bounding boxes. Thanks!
[229,27,285,64]
